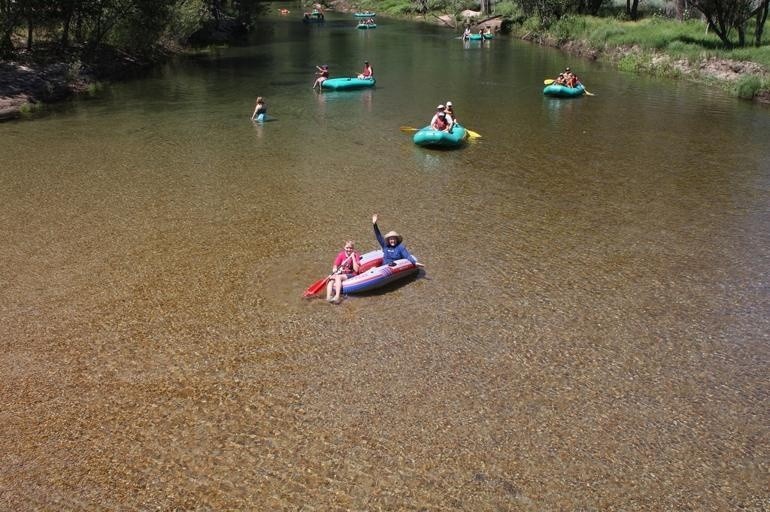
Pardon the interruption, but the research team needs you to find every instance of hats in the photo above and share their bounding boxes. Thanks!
[439,112,445,116]
[383,231,402,245]
[437,105,445,109]
[447,101,452,106]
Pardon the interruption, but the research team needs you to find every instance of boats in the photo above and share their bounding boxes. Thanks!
[544,82,585,96]
[354,13,376,17]
[321,77,375,89]
[468,33,493,40]
[328,248,418,295]
[413,125,467,146]
[358,23,377,29]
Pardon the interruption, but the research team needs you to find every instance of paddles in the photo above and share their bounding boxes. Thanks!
[399,126,431,134]
[303,255,351,298]
[578,82,595,97]
[543,79,556,85]
[456,123,482,139]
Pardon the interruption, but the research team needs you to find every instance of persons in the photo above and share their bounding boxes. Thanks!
[483,26,490,35]
[462,24,471,41]
[358,10,375,25]
[313,64,329,92]
[479,28,485,43]
[372,213,425,268]
[357,60,374,80]
[251,96,266,120]
[325,240,361,303]
[431,100,457,134]
[552,67,580,88]
[302,2,325,22]
